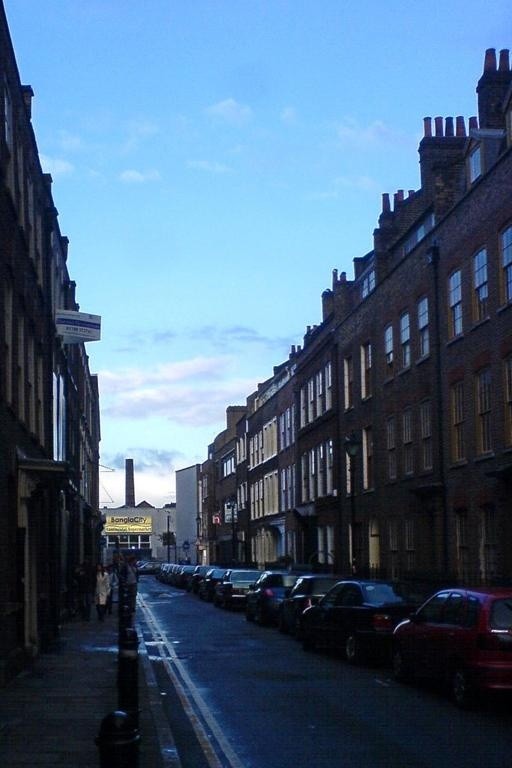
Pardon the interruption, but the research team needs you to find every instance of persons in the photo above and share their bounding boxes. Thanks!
[93,562,119,622]
[119,557,140,614]
[74,560,93,621]
